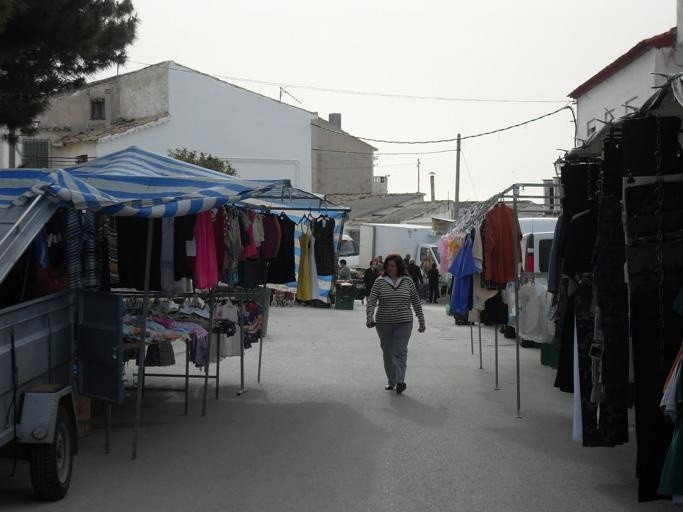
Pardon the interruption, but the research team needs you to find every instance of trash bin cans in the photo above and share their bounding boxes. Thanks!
[334,283,355,310]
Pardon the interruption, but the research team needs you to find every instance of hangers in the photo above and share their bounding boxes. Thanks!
[297,205,330,227]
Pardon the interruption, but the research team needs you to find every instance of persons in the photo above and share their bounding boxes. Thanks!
[366,252,441,394]
[338,259,351,303]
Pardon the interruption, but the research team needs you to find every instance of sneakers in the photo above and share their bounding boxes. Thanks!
[396,383,406,393]
[385,384,395,389]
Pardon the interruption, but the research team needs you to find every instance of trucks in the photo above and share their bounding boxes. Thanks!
[360,222,451,295]
[1,167,80,500]
